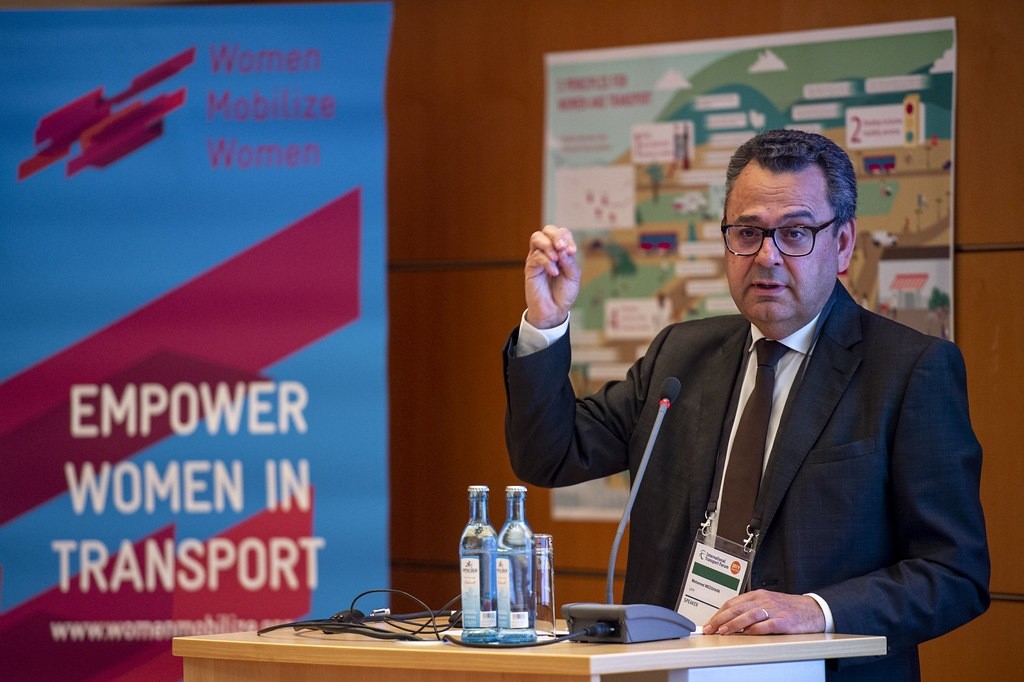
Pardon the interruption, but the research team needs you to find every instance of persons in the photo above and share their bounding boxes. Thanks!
[500,129,991,682]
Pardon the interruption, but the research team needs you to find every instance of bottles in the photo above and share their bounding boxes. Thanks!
[497,485,538,643]
[459,486,499,644]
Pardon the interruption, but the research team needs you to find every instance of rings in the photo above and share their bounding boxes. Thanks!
[762,609,769,619]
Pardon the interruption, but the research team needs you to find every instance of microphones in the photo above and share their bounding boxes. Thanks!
[559,377,697,644]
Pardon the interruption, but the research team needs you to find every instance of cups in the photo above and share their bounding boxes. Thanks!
[532,534,557,642]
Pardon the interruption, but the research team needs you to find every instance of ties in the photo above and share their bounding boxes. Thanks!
[714,339,790,594]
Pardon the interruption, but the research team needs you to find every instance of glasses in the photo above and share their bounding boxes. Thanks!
[720,217,836,258]
